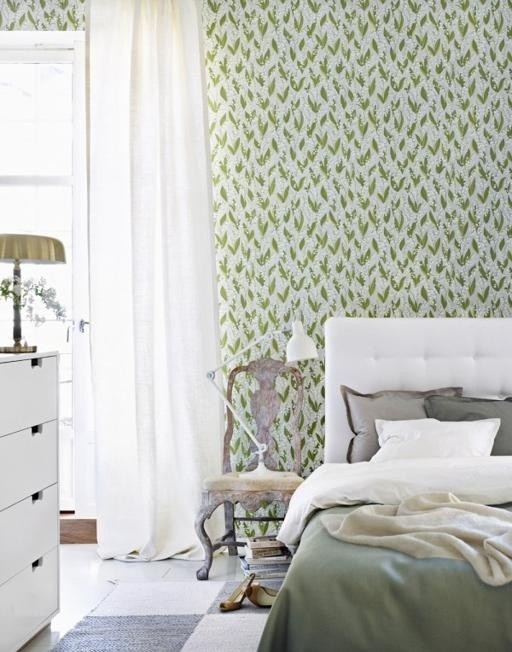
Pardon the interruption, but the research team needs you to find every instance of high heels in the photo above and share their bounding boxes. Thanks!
[245,585,279,608]
[220,573,260,612]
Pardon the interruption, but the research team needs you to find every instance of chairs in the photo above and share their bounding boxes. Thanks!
[191,356,308,579]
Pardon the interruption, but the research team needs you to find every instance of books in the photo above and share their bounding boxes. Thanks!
[238,535,291,581]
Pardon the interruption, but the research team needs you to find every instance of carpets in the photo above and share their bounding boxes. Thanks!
[51,574,283,650]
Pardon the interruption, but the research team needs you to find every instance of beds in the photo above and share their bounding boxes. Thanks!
[266,313,512,651]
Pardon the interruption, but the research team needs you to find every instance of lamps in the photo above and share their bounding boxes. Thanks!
[0,230,69,354]
[205,318,318,477]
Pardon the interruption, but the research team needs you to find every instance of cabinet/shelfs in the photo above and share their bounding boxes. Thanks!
[0,353,63,649]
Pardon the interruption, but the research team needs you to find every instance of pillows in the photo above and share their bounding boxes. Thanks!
[332,382,512,463]
